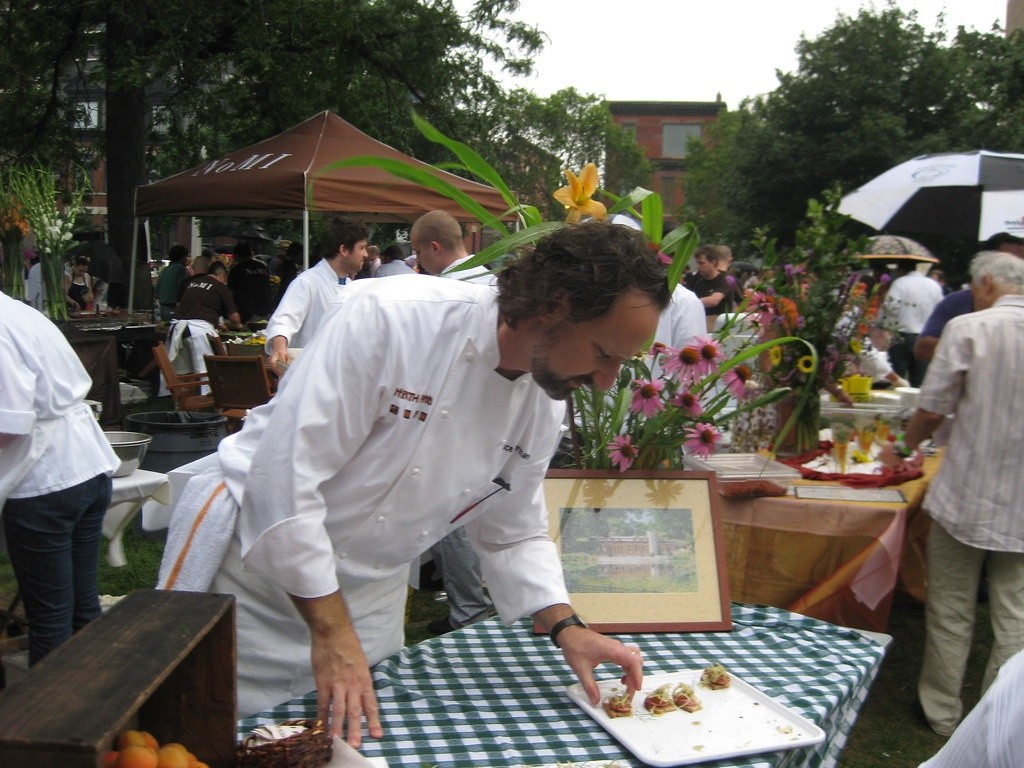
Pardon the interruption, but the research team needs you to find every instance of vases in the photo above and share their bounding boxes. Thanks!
[766,389,819,452]
[41,241,70,321]
[1,236,26,302]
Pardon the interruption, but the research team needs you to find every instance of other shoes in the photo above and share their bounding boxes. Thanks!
[429,615,456,636]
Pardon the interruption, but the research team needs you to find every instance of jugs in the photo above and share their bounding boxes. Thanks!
[837,376,872,404]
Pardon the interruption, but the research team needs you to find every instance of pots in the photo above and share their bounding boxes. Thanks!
[246,322,267,332]
[226,341,271,365]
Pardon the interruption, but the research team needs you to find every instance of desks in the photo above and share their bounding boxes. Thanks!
[101,471,169,567]
[810,378,951,447]
[717,334,892,389]
[698,444,940,628]
[715,306,859,333]
[237,596,896,767]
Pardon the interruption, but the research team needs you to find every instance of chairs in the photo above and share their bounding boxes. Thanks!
[150,332,286,431]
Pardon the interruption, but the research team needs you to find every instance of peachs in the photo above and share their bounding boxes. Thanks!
[104,730,210,768]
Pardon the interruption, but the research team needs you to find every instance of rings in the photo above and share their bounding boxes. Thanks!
[632,650,637,655]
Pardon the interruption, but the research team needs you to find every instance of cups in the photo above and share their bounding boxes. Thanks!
[830,419,891,475]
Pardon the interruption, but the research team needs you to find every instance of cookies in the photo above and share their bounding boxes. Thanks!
[604,682,731,718]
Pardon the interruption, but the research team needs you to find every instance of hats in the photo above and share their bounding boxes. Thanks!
[577,214,672,265]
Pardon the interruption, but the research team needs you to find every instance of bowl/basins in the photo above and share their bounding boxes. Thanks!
[99,431,152,478]
[897,387,921,405]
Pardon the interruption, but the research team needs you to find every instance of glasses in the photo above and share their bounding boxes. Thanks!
[450,477,510,525]
[76,256,91,263]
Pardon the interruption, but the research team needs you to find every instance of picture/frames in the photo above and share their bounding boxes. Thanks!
[527,471,732,633]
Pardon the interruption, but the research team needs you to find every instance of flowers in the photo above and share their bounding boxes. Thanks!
[0,183,33,247]
[300,84,819,470]
[710,197,876,389]
[12,159,97,249]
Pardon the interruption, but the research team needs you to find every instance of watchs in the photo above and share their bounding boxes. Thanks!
[551,613,588,649]
[893,432,911,458]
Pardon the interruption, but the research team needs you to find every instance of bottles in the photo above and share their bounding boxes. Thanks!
[154,299,161,322]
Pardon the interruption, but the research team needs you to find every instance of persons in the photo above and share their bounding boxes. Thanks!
[910,231,1024,603]
[151,222,672,751]
[63,257,95,314]
[0,290,122,670]
[655,244,772,348]
[832,258,954,389]
[917,649,1024,768]
[158,207,503,634]
[881,248,1024,736]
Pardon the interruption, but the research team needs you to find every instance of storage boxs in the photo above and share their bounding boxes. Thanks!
[0,586,238,768]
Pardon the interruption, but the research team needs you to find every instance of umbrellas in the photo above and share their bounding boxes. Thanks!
[824,142,1024,242]
[838,230,939,263]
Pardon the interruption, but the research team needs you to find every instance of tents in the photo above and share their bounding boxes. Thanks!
[126,109,518,313]
